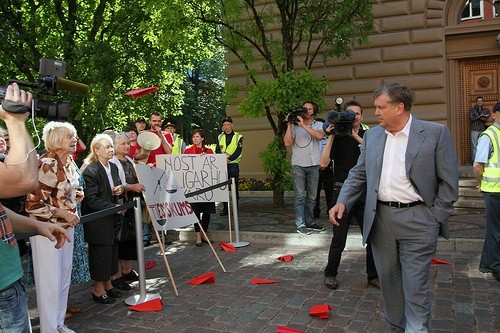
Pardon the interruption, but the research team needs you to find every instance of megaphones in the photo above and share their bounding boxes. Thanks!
[136,131,162,164]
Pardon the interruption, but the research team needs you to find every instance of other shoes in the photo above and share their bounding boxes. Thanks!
[202,236,214,245]
[195,239,202,246]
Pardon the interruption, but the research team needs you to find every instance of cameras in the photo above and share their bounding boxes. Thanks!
[0,57,69,122]
[288,103,308,125]
[322,95,356,136]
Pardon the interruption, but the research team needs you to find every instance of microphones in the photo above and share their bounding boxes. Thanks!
[41,75,89,95]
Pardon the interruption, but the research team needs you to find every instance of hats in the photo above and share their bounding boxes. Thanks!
[162,117,178,129]
[222,117,234,125]
[135,116,147,125]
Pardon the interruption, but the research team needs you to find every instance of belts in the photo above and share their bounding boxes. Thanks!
[376,198,428,209]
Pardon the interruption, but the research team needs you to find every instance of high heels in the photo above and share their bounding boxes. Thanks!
[105,287,122,298]
[91,289,116,304]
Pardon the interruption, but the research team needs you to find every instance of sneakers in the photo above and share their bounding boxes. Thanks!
[324,273,340,290]
[367,276,380,288]
[307,223,326,234]
[122,269,139,281]
[157,236,173,245]
[479,261,500,282]
[142,239,150,247]
[297,226,311,236]
[113,278,131,293]
[58,324,77,333]
[62,303,82,320]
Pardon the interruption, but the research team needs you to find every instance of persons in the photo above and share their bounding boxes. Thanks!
[472,102,500,282]
[182,128,215,248]
[283,101,335,236]
[320,100,382,290]
[0,82,186,333]
[327,82,459,333]
[468,96,490,166]
[215,116,241,216]
